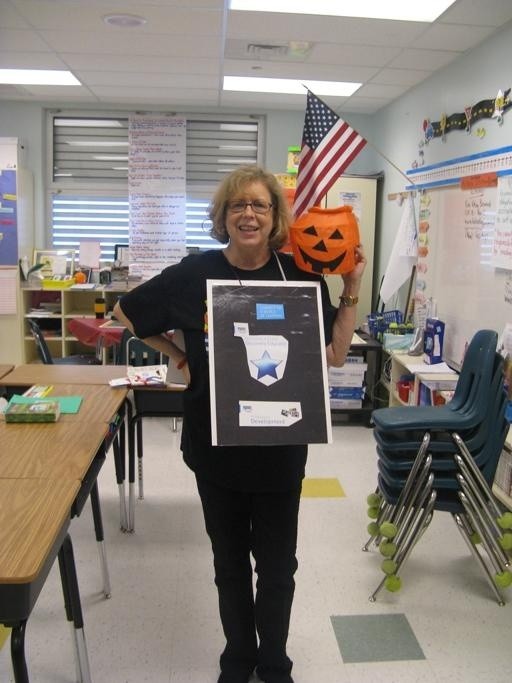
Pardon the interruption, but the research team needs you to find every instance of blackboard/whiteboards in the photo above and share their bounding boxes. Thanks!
[406,167,512,375]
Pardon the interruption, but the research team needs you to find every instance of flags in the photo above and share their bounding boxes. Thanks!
[289,88,367,219]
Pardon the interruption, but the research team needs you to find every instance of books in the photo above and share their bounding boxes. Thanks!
[402,358,460,408]
[70,268,143,292]
[24,290,62,317]
[8,392,82,415]
[5,399,61,422]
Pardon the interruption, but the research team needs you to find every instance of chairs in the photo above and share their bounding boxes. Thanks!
[24,317,173,532]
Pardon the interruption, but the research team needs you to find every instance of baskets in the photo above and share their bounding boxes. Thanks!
[366,309,414,343]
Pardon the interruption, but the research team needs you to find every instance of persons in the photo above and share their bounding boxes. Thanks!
[111,165,366,682]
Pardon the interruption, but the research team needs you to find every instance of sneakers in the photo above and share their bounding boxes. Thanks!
[258,664,298,683]
[215,653,256,683]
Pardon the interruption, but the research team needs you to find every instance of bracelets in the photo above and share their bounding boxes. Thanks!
[176,357,189,371]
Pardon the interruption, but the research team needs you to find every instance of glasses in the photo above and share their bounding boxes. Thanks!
[226,198,276,214]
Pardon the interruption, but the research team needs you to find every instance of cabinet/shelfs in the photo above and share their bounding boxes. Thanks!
[18,281,187,390]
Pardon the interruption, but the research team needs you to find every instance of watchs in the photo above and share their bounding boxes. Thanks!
[338,289,356,308]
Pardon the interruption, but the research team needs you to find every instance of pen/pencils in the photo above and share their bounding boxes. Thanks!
[22,383,53,399]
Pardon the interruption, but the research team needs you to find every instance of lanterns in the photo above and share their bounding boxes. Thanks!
[288,206,360,275]
